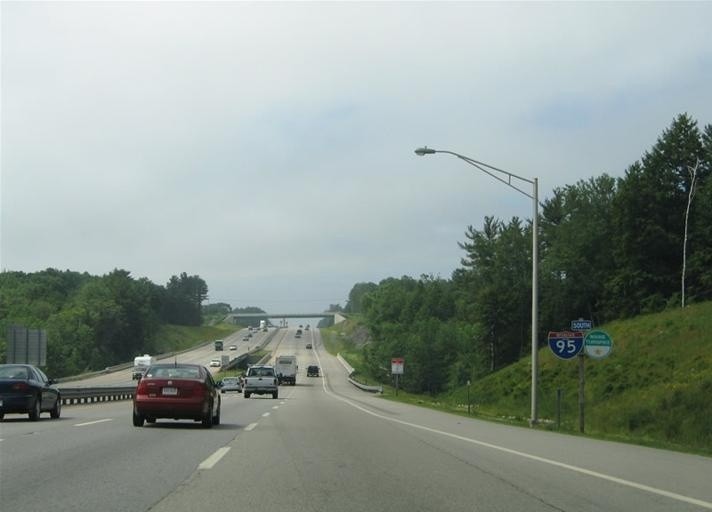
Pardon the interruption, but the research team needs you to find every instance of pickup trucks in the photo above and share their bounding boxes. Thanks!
[243,366,278,399]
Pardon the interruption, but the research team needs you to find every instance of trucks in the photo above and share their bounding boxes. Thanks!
[276,354,298,386]
[259,320,266,327]
[215,340,224,352]
[132,355,152,371]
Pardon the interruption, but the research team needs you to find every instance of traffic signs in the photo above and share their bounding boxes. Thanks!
[548,330,584,361]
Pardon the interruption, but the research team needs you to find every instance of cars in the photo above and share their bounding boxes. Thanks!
[131,355,223,429]
[0,365,62,422]
[295,321,313,350]
[209,359,221,369]
[307,366,320,377]
[132,366,148,380]
[230,325,268,351]
[219,377,241,393]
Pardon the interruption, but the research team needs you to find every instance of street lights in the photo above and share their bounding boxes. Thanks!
[414,147,541,427]
